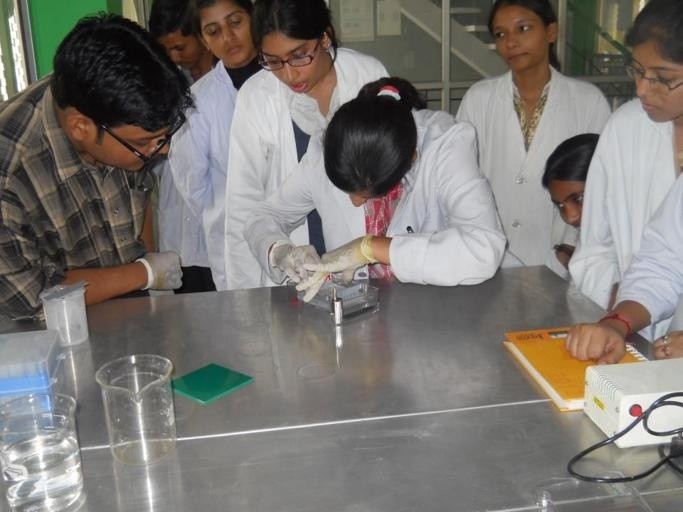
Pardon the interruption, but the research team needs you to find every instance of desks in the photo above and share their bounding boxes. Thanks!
[0,261,682,510]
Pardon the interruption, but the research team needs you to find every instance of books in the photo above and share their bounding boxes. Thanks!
[501,327,650,412]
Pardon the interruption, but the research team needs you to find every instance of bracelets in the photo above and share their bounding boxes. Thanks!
[602,314,632,336]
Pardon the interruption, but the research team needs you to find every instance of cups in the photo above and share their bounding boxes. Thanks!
[1,391,86,512]
[37,285,88,350]
[95,355,177,468]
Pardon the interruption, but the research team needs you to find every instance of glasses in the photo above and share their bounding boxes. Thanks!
[91,109,185,166]
[255,37,324,72]
[624,61,683,94]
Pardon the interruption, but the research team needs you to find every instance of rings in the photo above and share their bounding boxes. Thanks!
[663,334,670,344]
[662,345,671,357]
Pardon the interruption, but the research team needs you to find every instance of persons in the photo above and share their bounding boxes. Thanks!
[242,77,508,303]
[0,12,196,324]
[455,0,612,285]
[224,0,391,291]
[567,0,683,343]
[168,0,266,292]
[564,172,683,364]
[541,133,600,231]
[140,0,220,294]
